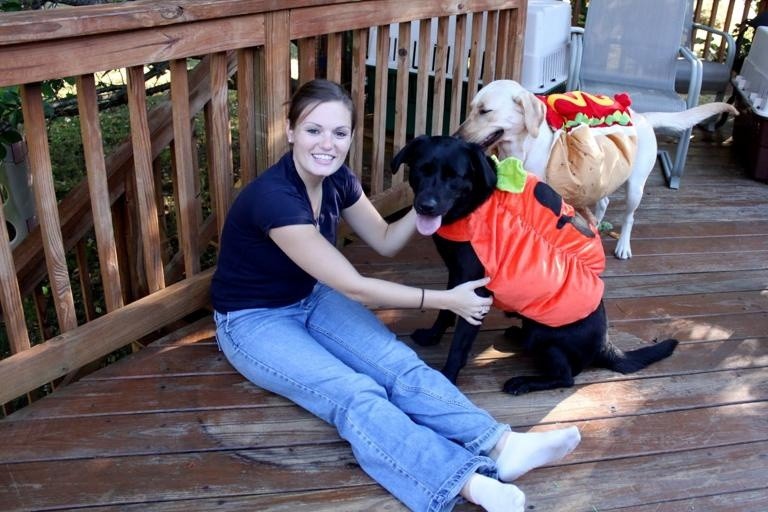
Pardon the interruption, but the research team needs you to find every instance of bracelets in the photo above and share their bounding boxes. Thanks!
[420,288,425,309]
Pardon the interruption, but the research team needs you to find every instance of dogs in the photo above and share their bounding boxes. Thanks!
[390,134,679,396]
[449,78,741,261]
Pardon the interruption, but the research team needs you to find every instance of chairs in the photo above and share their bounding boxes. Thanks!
[567,0,740,193]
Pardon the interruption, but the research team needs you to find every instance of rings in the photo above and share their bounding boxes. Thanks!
[481,306,487,316]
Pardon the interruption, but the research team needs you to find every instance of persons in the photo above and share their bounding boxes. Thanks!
[210,80,581,512]
